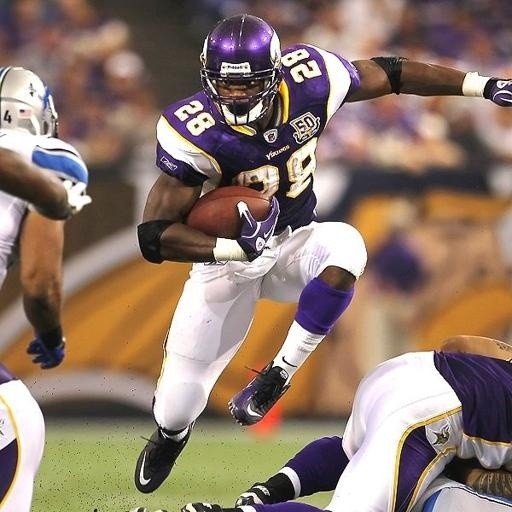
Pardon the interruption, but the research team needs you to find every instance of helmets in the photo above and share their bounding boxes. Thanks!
[200,16,284,126]
[0,66,60,142]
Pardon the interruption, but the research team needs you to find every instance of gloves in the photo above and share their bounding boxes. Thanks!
[25,330,66,369]
[232,196,280,264]
[24,174,93,221]
[482,72,512,107]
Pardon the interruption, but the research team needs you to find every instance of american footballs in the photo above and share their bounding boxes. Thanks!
[186,186,271,240]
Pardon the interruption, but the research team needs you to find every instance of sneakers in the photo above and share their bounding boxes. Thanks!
[134,424,193,494]
[226,357,293,427]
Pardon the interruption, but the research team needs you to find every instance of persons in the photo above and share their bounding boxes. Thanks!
[1,1,511,204]
[2,145,91,220]
[0,66,91,511]
[178,334,511,512]
[408,454,511,512]
[133,13,510,496]
